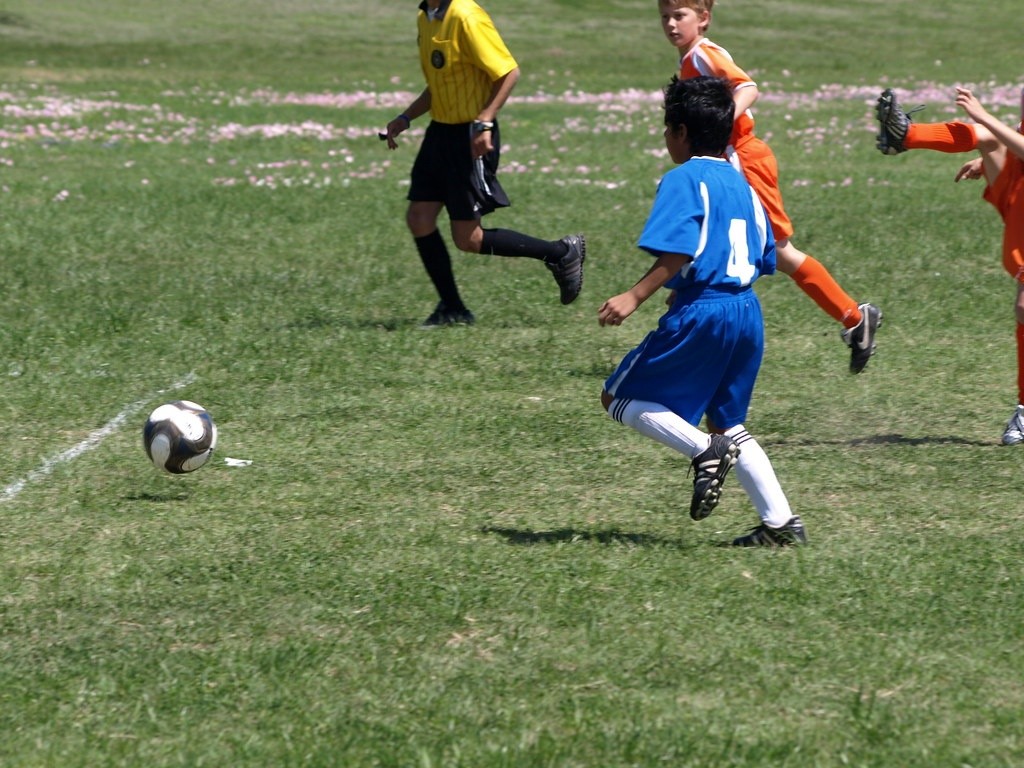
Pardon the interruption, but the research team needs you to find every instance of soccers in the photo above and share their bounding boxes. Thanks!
[142,399,220,476]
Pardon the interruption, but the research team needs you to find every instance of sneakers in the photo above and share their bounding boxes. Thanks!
[421,308,475,328]
[841,303,883,376]
[686,432,741,522]
[1002,405,1024,445]
[720,515,807,549]
[876,89,926,155]
[546,234,585,305]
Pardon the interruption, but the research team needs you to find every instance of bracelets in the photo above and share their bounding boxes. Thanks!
[399,113,410,129]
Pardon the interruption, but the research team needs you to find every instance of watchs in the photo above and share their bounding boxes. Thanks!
[471,117,494,132]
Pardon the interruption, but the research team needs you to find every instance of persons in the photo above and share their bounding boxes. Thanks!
[597,75,809,551]
[659,0,885,377]
[384,0,586,324]
[875,88,1024,445]
